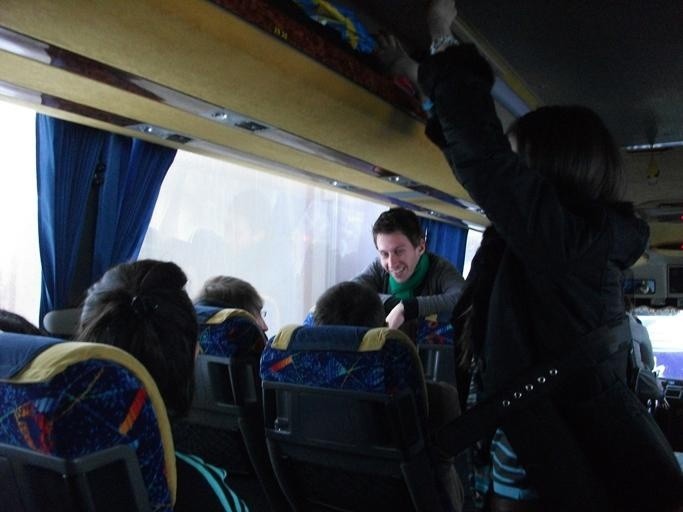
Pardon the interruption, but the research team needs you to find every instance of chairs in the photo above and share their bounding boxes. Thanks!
[0,328,179,512]
[304,305,317,325]
[194,303,288,511]
[259,327,468,512]
[407,311,457,383]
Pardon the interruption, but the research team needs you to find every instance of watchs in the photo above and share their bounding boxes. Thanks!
[430,33,460,54]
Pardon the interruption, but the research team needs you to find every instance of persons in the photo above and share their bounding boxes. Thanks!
[639,279,650,294]
[397,0,650,512]
[312,280,388,328]
[351,206,466,330]
[626,296,671,410]
[77,258,249,512]
[196,275,268,333]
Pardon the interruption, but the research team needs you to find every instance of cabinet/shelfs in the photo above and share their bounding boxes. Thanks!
[97,0,493,229]
